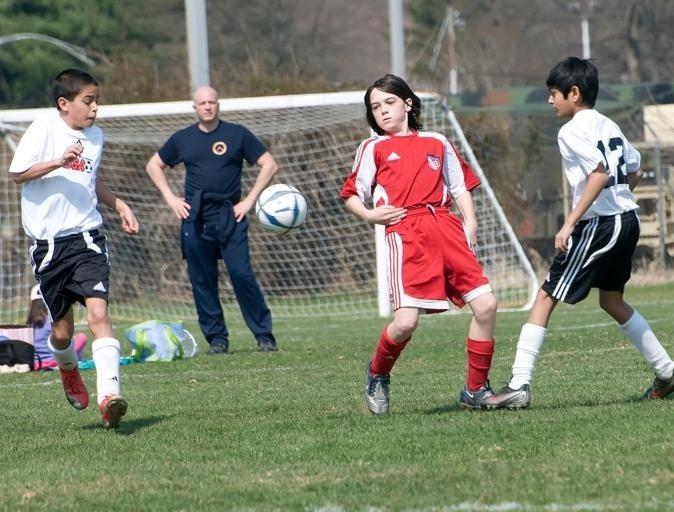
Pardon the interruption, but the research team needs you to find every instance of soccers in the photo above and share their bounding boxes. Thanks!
[256,184,307,235]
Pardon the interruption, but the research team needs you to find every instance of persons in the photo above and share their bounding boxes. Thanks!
[141,84,278,355]
[23,283,88,370]
[6,68,138,429]
[476,55,674,411]
[339,72,500,417]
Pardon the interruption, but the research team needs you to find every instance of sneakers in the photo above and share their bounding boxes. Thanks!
[458,380,532,410]
[57,364,90,411]
[642,369,674,400]
[205,343,227,355]
[257,341,278,351]
[97,392,130,431]
[364,359,394,417]
